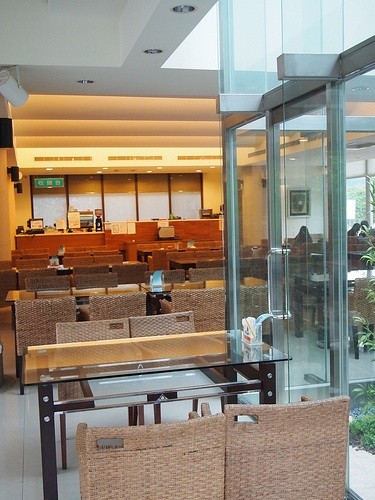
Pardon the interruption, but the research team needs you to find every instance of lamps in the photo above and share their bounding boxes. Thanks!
[7,166,19,182]
[14,183,23,194]
[0,118,14,149]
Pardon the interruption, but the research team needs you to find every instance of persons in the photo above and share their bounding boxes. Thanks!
[348,223,360,236]
[360,221,369,236]
[295,226,313,243]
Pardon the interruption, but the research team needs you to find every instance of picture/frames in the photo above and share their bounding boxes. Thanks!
[287,188,311,218]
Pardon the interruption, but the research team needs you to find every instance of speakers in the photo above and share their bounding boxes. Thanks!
[0,117,13,148]
[7,165,19,181]
[14,183,22,193]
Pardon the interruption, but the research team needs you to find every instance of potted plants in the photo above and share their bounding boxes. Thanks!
[343,246,375,500]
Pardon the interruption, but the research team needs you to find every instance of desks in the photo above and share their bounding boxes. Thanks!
[137,247,219,263]
[21,329,292,500]
[140,276,267,311]
[291,269,375,340]
[170,256,268,278]
[5,283,149,330]
[347,250,371,268]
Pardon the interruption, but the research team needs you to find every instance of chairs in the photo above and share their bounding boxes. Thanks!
[13,235,375,500]
